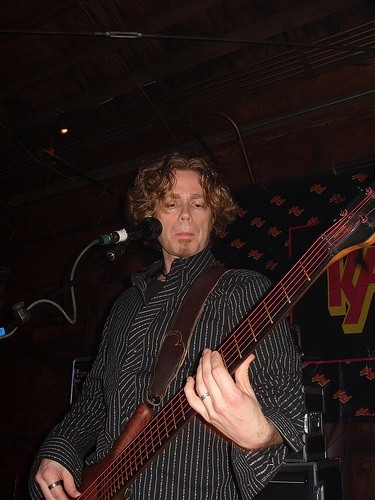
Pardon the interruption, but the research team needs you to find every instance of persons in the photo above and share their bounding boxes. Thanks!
[28,151,306,500]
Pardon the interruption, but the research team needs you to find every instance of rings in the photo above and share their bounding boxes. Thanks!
[200,393,210,401]
[48,481,61,490]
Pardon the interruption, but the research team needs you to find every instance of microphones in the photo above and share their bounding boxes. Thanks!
[99,217,163,246]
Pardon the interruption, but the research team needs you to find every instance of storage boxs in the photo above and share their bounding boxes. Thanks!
[284,433,328,463]
[253,457,344,500]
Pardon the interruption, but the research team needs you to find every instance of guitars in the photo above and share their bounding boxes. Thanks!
[74,180,375,500]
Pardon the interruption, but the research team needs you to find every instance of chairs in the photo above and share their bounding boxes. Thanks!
[0,431,40,500]
